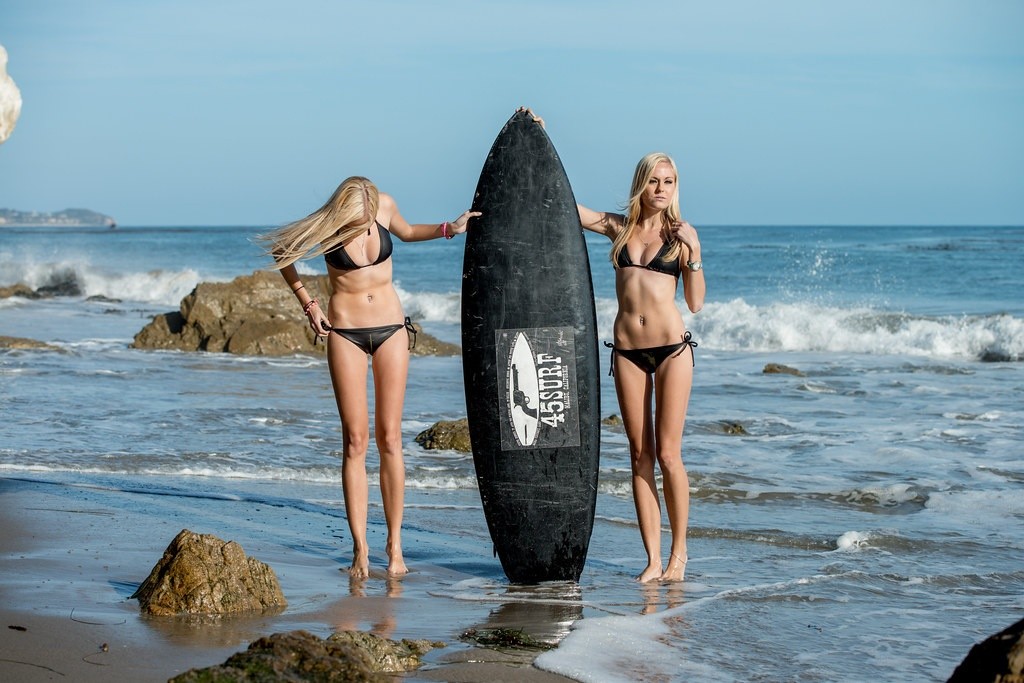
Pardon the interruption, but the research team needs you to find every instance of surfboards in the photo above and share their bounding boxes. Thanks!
[460,109,601,583]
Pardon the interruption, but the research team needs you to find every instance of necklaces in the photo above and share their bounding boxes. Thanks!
[638,223,663,248]
[353,231,366,256]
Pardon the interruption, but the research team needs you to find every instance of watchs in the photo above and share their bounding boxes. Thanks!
[687,260,703,272]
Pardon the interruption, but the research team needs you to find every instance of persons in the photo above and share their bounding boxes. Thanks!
[253,176,482,579]
[515,106,706,582]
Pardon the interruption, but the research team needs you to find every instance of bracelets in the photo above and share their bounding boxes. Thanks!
[440,221,455,240]
[302,299,319,316]
[293,286,305,294]
